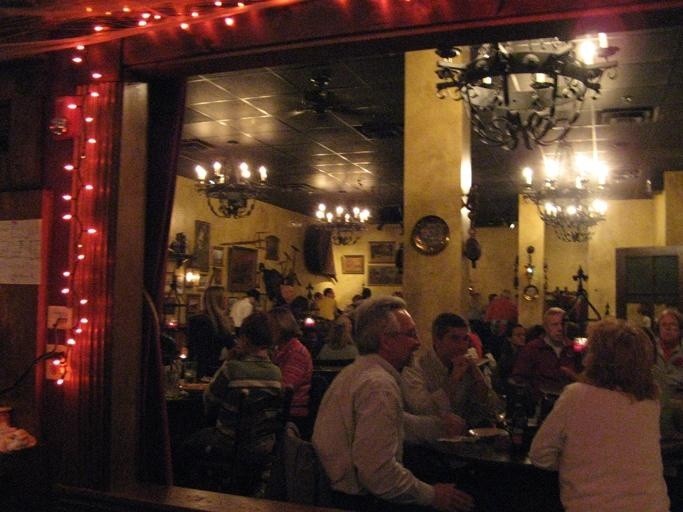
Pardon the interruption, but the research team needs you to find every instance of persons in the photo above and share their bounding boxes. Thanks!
[179,333,221,426]
[514,307,590,424]
[479,294,497,332]
[308,293,322,313]
[632,301,655,334]
[553,287,560,305]
[507,325,529,358]
[229,288,259,335]
[316,288,339,324]
[480,318,512,362]
[563,287,569,308]
[246,296,272,349]
[529,317,671,510]
[651,309,682,401]
[500,324,536,425]
[464,335,479,365]
[316,316,358,388]
[204,312,282,492]
[257,306,314,442]
[463,331,482,362]
[576,285,588,319]
[291,296,312,324]
[486,289,518,334]
[187,286,237,363]
[309,293,476,511]
[402,312,502,486]
[357,288,372,306]
[345,295,360,313]
[151,321,176,366]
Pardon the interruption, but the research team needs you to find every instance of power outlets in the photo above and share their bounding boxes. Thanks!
[45,343,69,382]
[46,305,72,331]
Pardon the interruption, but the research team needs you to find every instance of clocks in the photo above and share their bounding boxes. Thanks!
[522,245,539,305]
[410,215,450,256]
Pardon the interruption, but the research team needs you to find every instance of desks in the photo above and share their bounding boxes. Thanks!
[530,376,566,427]
[429,426,564,472]
[163,372,220,406]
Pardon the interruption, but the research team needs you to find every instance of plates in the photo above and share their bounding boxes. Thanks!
[183,383,205,397]
[468,424,506,441]
[437,436,470,449]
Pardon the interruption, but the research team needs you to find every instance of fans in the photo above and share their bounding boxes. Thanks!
[270,69,373,130]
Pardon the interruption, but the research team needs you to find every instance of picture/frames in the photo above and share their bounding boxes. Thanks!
[341,254,365,275]
[367,240,398,264]
[367,264,403,288]
[191,219,211,277]
[227,245,260,293]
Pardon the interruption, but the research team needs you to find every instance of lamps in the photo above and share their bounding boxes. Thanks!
[540,201,610,241]
[313,202,377,245]
[194,161,270,219]
[434,31,620,150]
[518,152,624,207]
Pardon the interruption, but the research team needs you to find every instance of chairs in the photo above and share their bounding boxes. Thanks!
[304,357,355,428]
[232,385,294,500]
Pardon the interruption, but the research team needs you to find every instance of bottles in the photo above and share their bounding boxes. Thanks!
[508,388,528,442]
